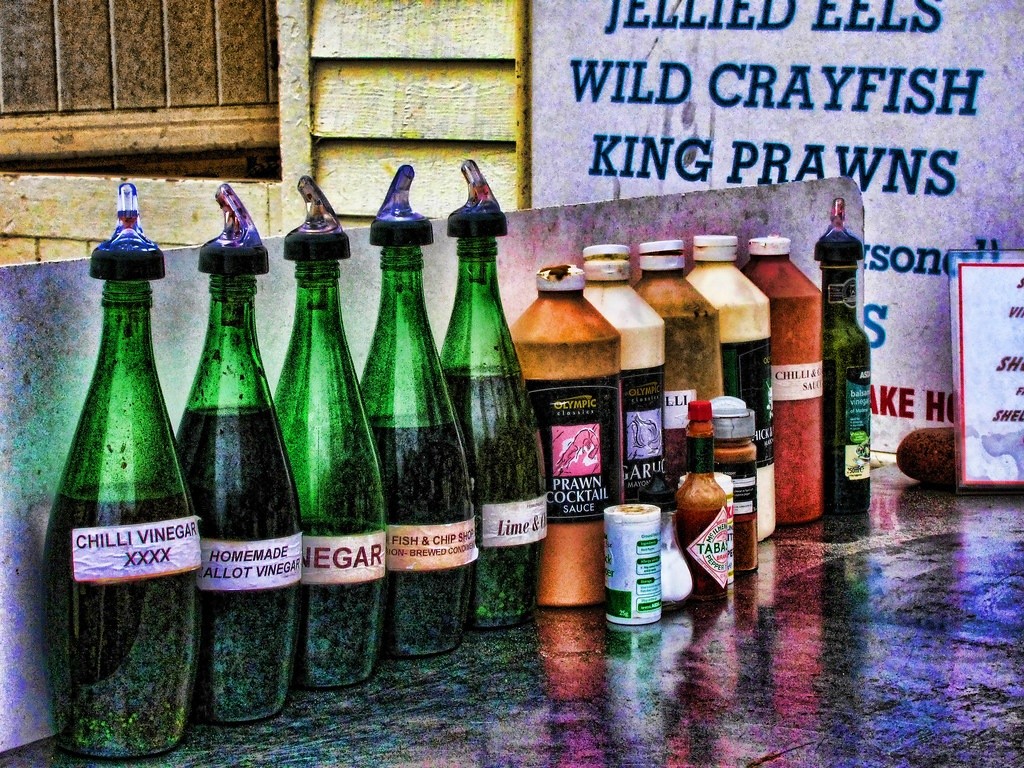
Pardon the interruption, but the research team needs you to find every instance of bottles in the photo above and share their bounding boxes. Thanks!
[41,183,201,758]
[360,164,479,658]
[639,469,692,607]
[506,264,625,609]
[273,175,386,691]
[810,197,872,518]
[580,243,666,509]
[738,234,823,523]
[631,238,724,489]
[601,501,660,625]
[676,469,739,590]
[672,401,730,599]
[173,183,302,725]
[687,234,777,540]
[703,392,761,570]
[436,158,548,629]
